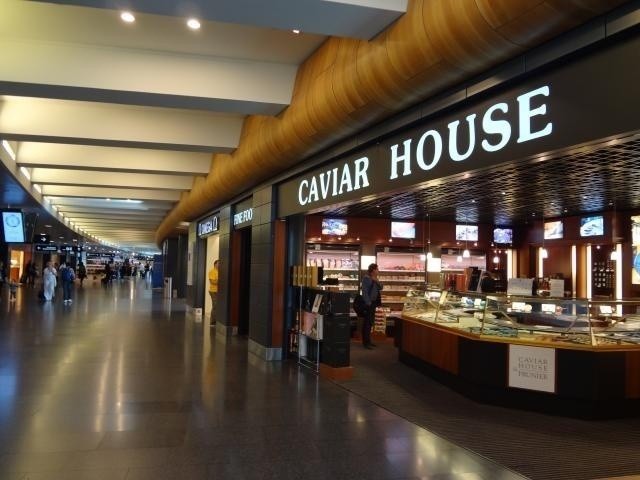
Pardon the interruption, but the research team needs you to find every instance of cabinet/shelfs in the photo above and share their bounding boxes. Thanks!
[306,243,426,328]
[399,285,640,418]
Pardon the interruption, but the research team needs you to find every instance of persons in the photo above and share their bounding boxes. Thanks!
[0,260,87,303]
[138,261,152,279]
[362,264,383,350]
[209,260,219,325]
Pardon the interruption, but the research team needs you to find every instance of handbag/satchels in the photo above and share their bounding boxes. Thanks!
[353,294,369,317]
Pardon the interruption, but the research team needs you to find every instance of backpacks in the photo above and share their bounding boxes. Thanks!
[62,267,71,281]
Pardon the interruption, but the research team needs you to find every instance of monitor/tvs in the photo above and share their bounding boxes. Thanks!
[455,224,479,241]
[494,228,513,244]
[321,218,348,236]
[544,221,564,240]
[391,222,416,239]
[579,215,604,236]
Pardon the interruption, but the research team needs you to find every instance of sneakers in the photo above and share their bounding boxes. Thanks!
[364,344,376,349]
[64,298,72,303]
